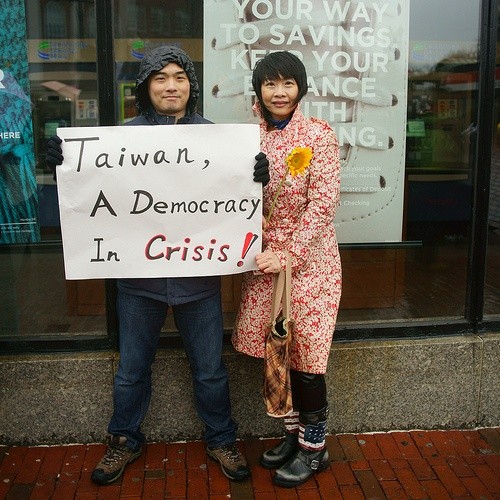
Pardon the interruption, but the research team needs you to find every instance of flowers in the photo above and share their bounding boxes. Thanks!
[267,146,312,220]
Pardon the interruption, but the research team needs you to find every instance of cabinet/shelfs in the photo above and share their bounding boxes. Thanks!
[407,0,500,183]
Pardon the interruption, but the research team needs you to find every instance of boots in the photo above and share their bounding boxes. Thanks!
[260,409,299,469]
[273,401,329,487]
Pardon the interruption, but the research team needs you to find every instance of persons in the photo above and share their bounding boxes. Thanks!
[44,45,271,485]
[230,51,343,488]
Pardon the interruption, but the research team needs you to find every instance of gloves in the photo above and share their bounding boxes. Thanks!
[45,135,64,171]
[253,152,270,188]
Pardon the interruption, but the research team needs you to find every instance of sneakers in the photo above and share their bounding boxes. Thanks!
[91,434,143,485]
[206,442,250,481]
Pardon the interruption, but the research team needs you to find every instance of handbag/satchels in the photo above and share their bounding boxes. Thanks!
[260,251,293,418]
